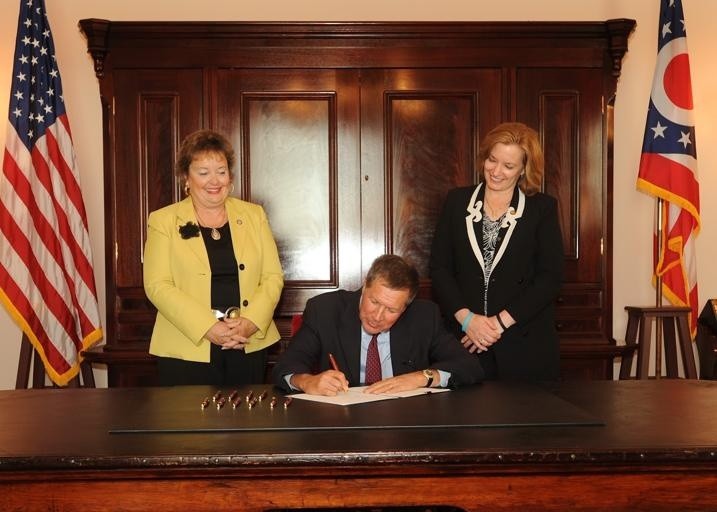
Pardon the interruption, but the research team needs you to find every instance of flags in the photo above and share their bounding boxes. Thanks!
[0,0,103,388]
[636,0,701,378]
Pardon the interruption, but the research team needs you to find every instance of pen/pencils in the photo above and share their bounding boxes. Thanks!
[328,352,338,371]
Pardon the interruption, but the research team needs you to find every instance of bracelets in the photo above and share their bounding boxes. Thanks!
[496,314,508,331]
[461,312,474,332]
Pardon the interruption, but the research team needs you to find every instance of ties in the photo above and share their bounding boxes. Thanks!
[365,332,382,385]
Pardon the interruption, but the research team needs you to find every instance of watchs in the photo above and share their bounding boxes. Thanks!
[422,369,434,387]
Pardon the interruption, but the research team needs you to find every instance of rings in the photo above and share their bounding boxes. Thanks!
[479,339,484,344]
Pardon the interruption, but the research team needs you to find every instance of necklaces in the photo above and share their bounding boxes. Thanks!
[487,199,511,218]
[196,206,225,240]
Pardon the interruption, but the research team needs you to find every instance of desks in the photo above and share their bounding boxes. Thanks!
[1,377,716,507]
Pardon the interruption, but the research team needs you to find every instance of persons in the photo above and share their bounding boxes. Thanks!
[430,122,563,382]
[271,255,484,398]
[142,129,285,386]
